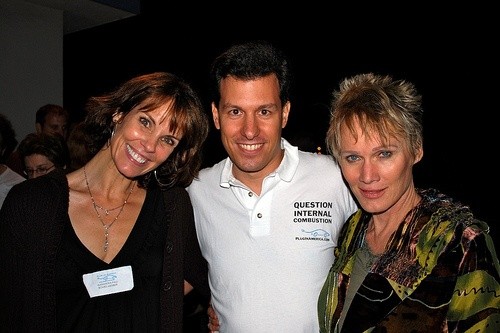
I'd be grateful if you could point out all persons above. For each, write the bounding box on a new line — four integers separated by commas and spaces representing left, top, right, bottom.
1, 71, 211, 332
35, 104, 68, 138
68, 123, 110, 171
16, 133, 67, 179
0, 114, 28, 209
208, 72, 500, 332
185, 47, 360, 332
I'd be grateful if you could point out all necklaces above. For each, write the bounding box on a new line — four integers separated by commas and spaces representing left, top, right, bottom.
83, 164, 136, 252
